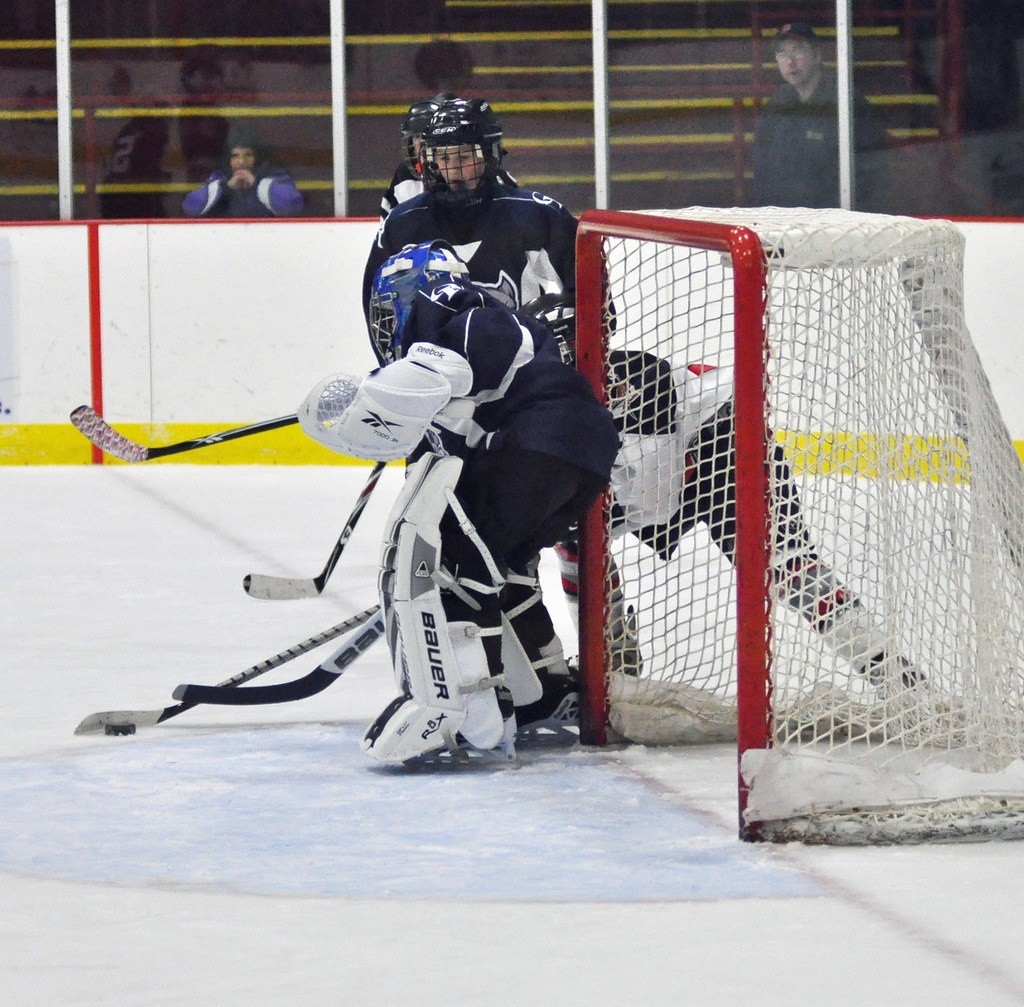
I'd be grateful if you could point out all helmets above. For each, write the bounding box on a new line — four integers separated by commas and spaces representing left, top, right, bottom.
368, 239, 469, 361
401, 91, 456, 180
419, 97, 503, 210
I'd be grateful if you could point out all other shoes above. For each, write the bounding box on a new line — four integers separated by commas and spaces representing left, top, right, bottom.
562, 605, 643, 676
866, 657, 923, 698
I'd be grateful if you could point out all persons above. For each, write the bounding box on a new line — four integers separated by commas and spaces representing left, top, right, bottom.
306, 240, 616, 764
522, 293, 930, 699
365, 92, 616, 386
753, 24, 887, 207
181, 125, 303, 219
100, 101, 173, 219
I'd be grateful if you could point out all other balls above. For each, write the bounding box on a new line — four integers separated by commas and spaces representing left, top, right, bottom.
104, 722, 137, 737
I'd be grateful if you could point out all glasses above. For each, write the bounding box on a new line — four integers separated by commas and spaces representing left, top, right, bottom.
775, 44, 810, 60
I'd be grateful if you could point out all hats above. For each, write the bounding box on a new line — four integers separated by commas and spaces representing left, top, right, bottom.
773, 21, 817, 48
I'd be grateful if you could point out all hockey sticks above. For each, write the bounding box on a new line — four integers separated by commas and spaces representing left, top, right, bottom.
239, 462, 386, 601
69, 403, 353, 463
171, 606, 388, 707
72, 602, 382, 738
